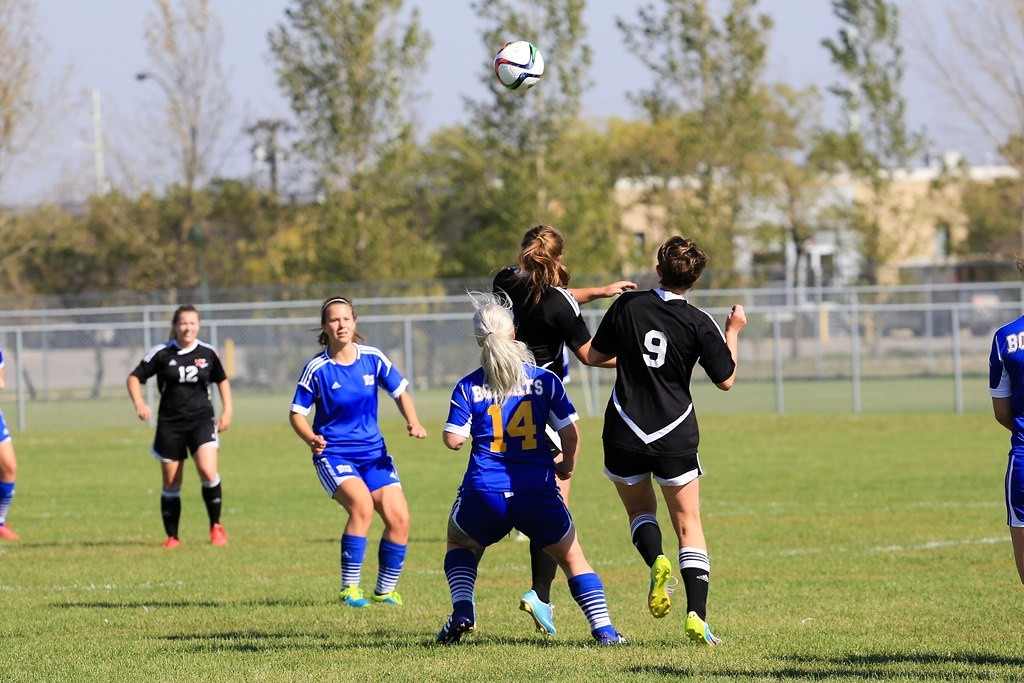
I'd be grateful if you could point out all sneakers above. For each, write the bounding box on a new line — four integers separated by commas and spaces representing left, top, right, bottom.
647, 555, 672, 620
0, 523, 21, 543
162, 536, 180, 550
436, 604, 474, 646
519, 590, 556, 641
595, 631, 630, 645
338, 584, 371, 608
371, 591, 403, 607
210, 523, 226, 546
683, 611, 723, 648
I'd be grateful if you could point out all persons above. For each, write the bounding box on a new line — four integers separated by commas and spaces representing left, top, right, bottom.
493, 225, 638, 636
289, 296, 427, 607
0, 350, 21, 541
989, 254, 1024, 585
588, 237, 747, 647
435, 293, 627, 646
127, 304, 232, 550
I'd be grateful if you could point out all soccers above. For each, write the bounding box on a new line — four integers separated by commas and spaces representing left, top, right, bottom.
492, 39, 546, 94
